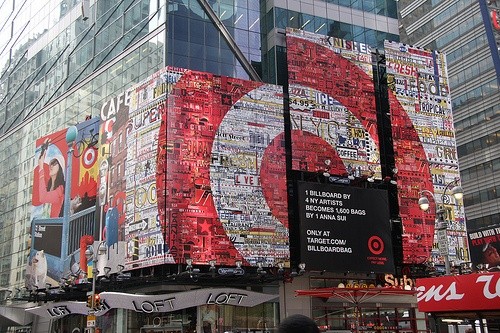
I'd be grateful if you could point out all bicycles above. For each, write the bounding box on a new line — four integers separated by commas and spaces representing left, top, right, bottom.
73, 128, 99, 158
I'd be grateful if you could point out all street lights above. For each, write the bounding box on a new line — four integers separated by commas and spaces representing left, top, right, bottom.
84, 239, 107, 333
58, 126, 78, 276
418, 177, 464, 276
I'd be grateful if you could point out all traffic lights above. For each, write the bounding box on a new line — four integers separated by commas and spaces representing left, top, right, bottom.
86, 293, 100, 311
